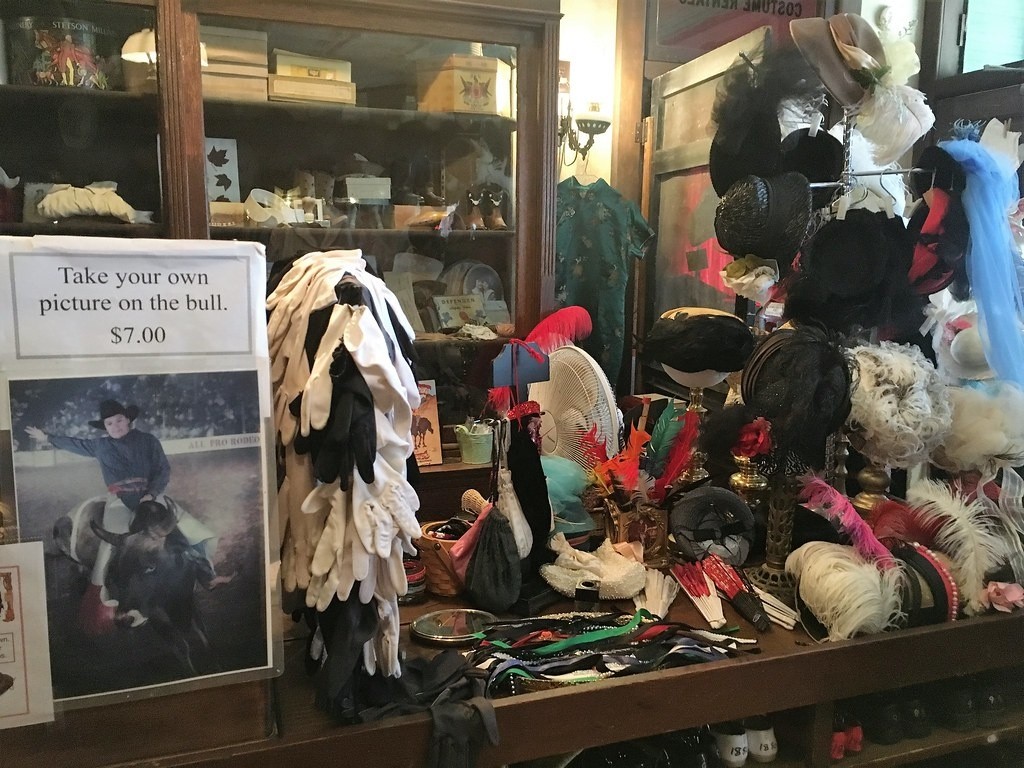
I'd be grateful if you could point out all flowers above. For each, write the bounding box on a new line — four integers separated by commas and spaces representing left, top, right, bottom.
879, 30, 921, 86
720, 253, 780, 304
941, 319, 972, 346
731, 418, 772, 460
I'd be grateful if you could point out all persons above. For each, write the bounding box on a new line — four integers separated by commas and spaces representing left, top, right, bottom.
23, 399, 237, 622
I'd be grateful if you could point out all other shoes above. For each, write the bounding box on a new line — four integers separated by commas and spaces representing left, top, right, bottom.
830, 705, 862, 761
663, 729, 725, 768
917, 677, 1008, 732
709, 716, 779, 768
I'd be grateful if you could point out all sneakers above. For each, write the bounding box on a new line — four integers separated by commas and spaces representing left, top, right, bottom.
847, 691, 932, 745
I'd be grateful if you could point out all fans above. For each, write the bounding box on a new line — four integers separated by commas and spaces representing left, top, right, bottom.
529, 346, 626, 472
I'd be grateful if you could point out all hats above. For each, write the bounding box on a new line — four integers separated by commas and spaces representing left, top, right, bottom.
708, 12, 954, 469
86, 399, 140, 430
670, 485, 756, 566
931, 300, 1024, 381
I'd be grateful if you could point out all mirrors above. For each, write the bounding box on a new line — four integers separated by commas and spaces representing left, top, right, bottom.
409, 608, 498, 645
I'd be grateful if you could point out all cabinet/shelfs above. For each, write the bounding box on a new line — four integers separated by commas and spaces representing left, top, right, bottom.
0, 0, 564, 519
0, 561, 1024, 768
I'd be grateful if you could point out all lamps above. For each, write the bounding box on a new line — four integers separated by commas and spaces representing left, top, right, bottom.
557, 89, 610, 162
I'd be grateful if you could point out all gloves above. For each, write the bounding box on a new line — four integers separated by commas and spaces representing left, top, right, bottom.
428, 674, 499, 768
360, 648, 469, 723
267, 248, 422, 679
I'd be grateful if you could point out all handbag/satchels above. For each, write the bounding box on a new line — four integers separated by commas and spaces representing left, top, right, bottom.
464, 507, 523, 614
489, 466, 533, 560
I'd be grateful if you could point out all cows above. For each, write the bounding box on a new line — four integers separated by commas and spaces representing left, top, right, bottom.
51, 494, 229, 692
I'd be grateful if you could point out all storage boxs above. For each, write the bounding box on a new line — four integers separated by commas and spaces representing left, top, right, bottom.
199, 25, 358, 106
345, 177, 392, 199
209, 201, 257, 228
383, 204, 447, 230
406, 53, 511, 117
